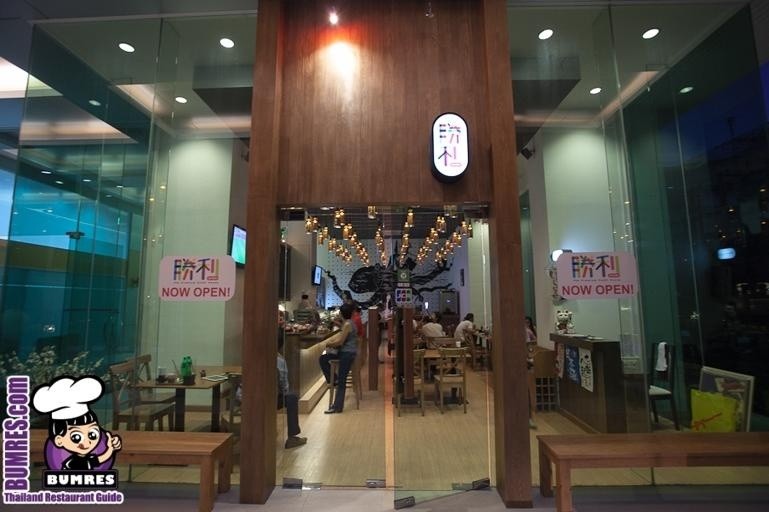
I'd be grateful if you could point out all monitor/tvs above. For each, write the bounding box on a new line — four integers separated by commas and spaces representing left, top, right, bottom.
229, 224, 246, 268
312, 264, 322, 286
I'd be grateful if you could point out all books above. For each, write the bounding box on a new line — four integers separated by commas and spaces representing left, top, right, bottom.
698, 365, 755, 433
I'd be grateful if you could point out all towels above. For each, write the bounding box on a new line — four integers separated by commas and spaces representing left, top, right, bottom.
655, 342, 668, 371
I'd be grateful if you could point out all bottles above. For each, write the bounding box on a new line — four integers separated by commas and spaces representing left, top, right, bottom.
181, 356, 192, 381
201, 370, 206, 377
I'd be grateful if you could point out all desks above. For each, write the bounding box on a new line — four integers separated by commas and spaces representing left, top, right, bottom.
131, 365, 243, 432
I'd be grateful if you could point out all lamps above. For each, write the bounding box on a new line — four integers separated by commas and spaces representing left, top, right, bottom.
305, 205, 473, 267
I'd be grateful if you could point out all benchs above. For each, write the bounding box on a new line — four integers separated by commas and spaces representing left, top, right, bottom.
29, 430, 234, 512
537, 431, 769, 512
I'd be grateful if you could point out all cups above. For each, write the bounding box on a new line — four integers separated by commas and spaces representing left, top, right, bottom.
167, 373, 176, 383
158, 366, 166, 383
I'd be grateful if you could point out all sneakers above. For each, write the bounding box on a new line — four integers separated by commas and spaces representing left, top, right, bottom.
324, 406, 342, 413
285, 436, 307, 448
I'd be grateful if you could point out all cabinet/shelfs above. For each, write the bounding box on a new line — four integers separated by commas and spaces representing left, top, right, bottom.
528, 372, 560, 412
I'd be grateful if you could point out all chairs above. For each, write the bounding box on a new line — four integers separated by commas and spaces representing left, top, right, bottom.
110, 362, 175, 431
221, 373, 242, 472
328, 329, 491, 417
129, 354, 178, 404
646, 343, 679, 431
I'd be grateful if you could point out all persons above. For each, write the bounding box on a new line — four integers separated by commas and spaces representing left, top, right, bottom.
524, 316, 537, 344
413, 314, 422, 331
232, 327, 308, 448
316, 303, 358, 414
419, 317, 446, 350
330, 299, 364, 348
452, 312, 475, 344
430, 311, 443, 326
